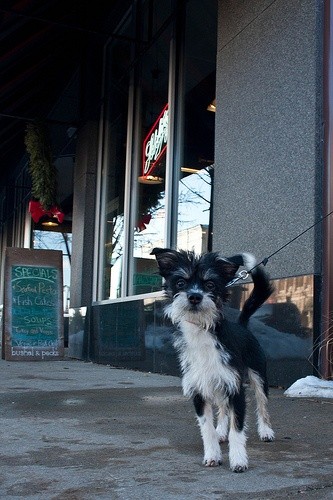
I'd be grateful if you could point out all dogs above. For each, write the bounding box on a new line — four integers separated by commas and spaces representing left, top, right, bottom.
149, 247, 276, 473
